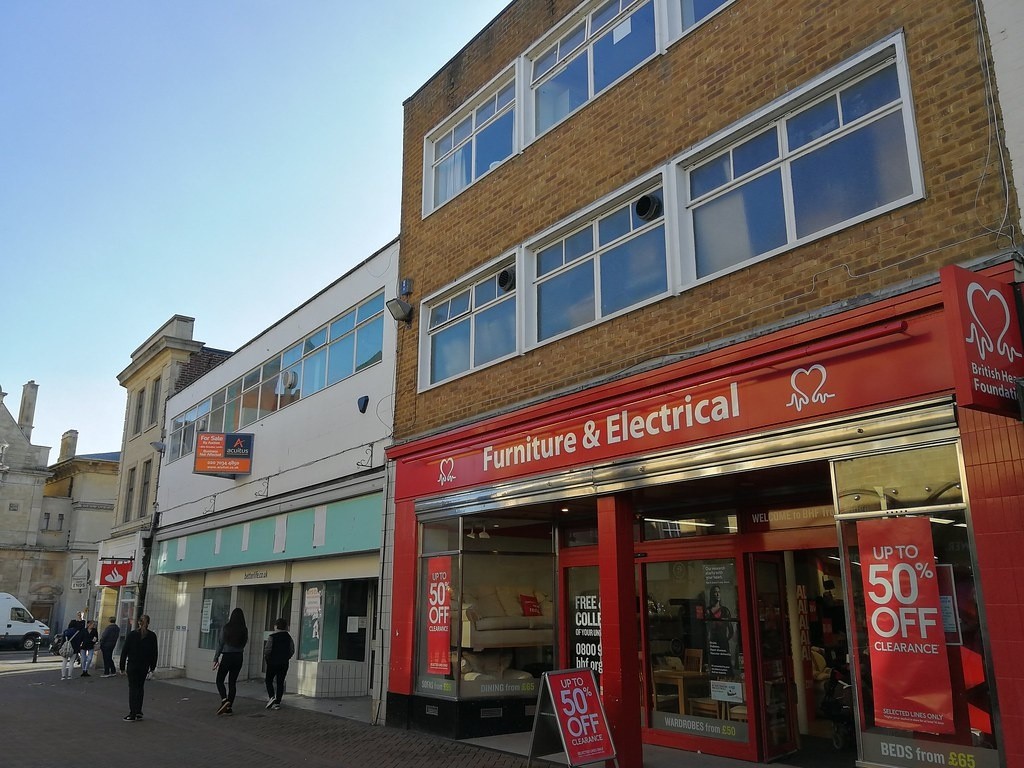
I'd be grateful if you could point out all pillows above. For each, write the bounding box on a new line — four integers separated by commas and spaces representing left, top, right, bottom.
519, 594, 544, 617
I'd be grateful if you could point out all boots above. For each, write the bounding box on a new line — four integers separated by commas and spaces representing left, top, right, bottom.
81, 670, 85, 677
86, 669, 91, 676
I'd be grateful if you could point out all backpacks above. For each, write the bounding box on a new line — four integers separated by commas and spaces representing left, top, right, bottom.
59, 630, 80, 658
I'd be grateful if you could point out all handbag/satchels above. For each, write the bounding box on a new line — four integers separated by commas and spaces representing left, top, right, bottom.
94, 642, 101, 651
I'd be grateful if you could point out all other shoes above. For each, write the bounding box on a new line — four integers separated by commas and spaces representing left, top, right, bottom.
67, 677, 72, 679
61, 677, 65, 680
99, 674, 109, 677
109, 674, 116, 676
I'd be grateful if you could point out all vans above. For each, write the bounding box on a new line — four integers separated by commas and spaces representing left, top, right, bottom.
0, 592, 51, 650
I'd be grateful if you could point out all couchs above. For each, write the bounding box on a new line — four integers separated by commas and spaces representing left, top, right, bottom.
450, 585, 553, 652
450, 651, 534, 681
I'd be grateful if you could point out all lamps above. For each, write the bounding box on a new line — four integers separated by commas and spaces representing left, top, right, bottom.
386, 298, 414, 324
466, 528, 476, 539
479, 529, 490, 539
149, 442, 165, 455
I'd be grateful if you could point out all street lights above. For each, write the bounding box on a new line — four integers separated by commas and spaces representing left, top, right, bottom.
124, 441, 167, 628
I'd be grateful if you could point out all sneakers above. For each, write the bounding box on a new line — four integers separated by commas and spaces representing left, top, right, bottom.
223, 708, 232, 715
215, 699, 231, 715
136, 714, 144, 720
123, 715, 135, 721
265, 696, 276, 708
271, 704, 280, 710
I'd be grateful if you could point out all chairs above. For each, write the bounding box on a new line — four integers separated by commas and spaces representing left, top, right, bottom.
637, 648, 747, 723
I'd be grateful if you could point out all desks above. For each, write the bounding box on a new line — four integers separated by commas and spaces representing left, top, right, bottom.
653, 669, 707, 716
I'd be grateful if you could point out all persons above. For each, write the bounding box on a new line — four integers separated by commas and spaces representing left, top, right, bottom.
264, 618, 295, 710
120, 615, 158, 721
212, 608, 248, 716
100, 616, 120, 678
59, 610, 98, 679
706, 585, 734, 677
